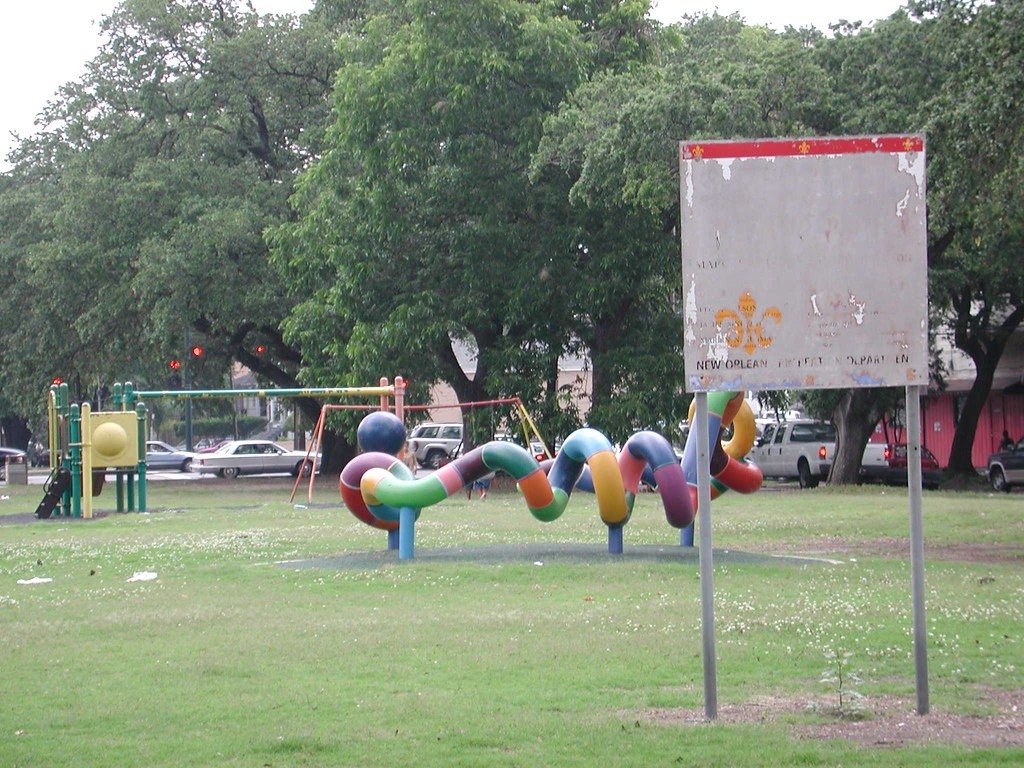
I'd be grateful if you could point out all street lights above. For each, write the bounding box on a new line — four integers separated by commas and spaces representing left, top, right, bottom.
168, 346, 203, 453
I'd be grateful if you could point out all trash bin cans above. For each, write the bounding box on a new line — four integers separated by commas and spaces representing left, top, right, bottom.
5, 455, 27, 485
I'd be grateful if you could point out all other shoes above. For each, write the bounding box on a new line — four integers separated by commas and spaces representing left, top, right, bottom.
480, 493, 486, 502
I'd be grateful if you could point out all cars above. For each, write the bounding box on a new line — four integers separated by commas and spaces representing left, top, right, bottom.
889, 443, 940, 470
0, 447, 28, 480
403, 421, 559, 471
646, 416, 891, 489
196, 439, 322, 480
987, 435, 1024, 493
144, 439, 200, 473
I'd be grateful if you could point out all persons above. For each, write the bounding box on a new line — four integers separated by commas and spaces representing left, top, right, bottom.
404, 442, 417, 475
459, 441, 495, 501
